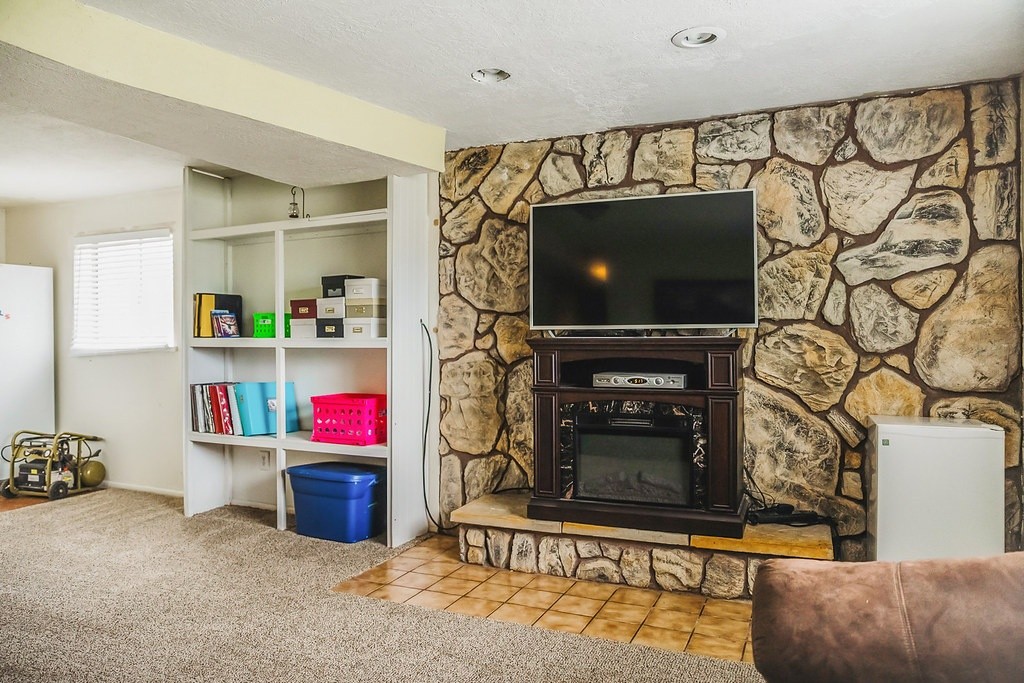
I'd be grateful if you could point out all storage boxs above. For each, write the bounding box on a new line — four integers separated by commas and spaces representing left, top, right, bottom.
286, 462, 386, 545
251, 312, 291, 338
288, 274, 385, 338
310, 393, 386, 445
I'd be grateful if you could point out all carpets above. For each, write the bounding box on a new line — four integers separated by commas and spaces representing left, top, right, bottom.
0, 487, 762, 683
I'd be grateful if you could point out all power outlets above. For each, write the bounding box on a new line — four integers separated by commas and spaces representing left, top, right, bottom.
259, 450, 270, 472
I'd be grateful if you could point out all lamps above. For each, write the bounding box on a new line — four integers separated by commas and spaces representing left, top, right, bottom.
288, 185, 307, 219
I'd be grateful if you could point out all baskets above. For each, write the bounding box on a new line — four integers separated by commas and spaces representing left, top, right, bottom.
310, 393, 387, 446
253, 312, 291, 338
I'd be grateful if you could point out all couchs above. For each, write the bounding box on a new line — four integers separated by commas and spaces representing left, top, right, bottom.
751, 550, 1024, 683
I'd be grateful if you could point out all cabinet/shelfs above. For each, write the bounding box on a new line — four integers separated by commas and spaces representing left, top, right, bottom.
183, 166, 440, 551
523, 337, 750, 539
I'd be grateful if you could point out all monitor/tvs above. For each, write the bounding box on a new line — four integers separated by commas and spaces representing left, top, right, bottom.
530, 188, 758, 338
